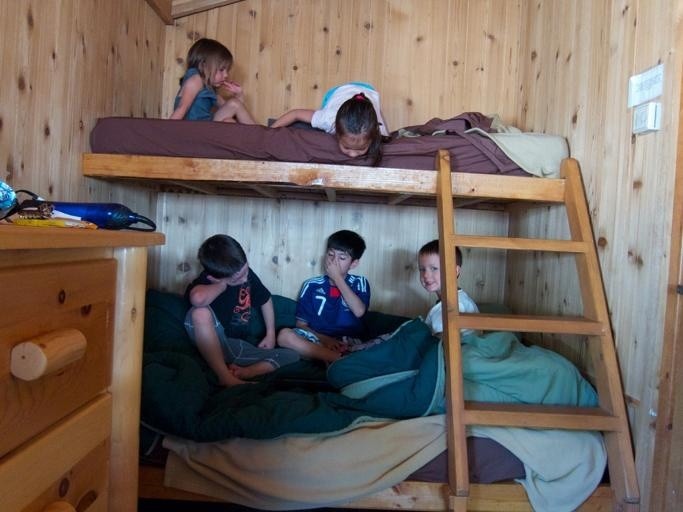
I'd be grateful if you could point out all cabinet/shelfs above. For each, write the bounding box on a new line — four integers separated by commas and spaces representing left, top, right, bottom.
0, 222, 168, 512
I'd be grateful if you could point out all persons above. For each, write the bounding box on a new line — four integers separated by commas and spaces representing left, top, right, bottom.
182, 233, 302, 389
347, 238, 484, 355
167, 37, 258, 126
268, 80, 392, 159
276, 228, 396, 365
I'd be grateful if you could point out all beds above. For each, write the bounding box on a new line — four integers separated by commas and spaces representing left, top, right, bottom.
82, 110, 640, 512
